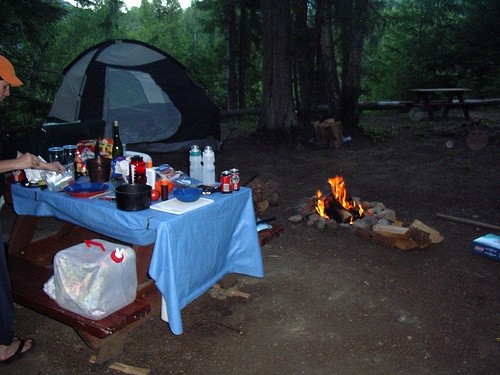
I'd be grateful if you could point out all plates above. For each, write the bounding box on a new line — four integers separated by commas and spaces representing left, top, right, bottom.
64, 183, 108, 197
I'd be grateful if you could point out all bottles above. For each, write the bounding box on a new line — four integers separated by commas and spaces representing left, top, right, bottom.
128, 154, 148, 184
112, 120, 123, 158
189, 145, 203, 181
202, 146, 216, 183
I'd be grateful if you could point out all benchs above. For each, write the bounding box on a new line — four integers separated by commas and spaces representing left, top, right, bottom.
399, 101, 477, 112
4, 252, 151, 339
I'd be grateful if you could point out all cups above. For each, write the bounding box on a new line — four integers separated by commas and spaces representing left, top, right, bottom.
63, 145, 79, 162
48, 147, 64, 164
86, 157, 112, 182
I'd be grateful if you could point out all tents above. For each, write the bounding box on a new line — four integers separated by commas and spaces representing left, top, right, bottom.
41, 37, 222, 154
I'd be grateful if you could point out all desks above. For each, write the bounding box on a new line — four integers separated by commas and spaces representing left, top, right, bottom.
4, 177, 266, 375
407, 88, 473, 121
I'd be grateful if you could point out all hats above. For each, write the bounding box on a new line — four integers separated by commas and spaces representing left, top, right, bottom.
0, 55, 23, 87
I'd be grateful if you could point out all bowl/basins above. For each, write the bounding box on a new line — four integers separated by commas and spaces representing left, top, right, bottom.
114, 183, 151, 211
172, 187, 201, 202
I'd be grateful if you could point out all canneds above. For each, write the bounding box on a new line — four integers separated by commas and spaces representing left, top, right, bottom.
220, 171, 233, 194
229, 168, 240, 191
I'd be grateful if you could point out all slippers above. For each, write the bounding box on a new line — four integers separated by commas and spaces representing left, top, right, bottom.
0, 338, 34, 363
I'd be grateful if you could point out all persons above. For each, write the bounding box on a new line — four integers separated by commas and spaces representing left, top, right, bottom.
0, 54, 64, 366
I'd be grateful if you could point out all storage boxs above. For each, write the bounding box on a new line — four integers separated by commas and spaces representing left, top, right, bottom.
471, 233, 500, 261
